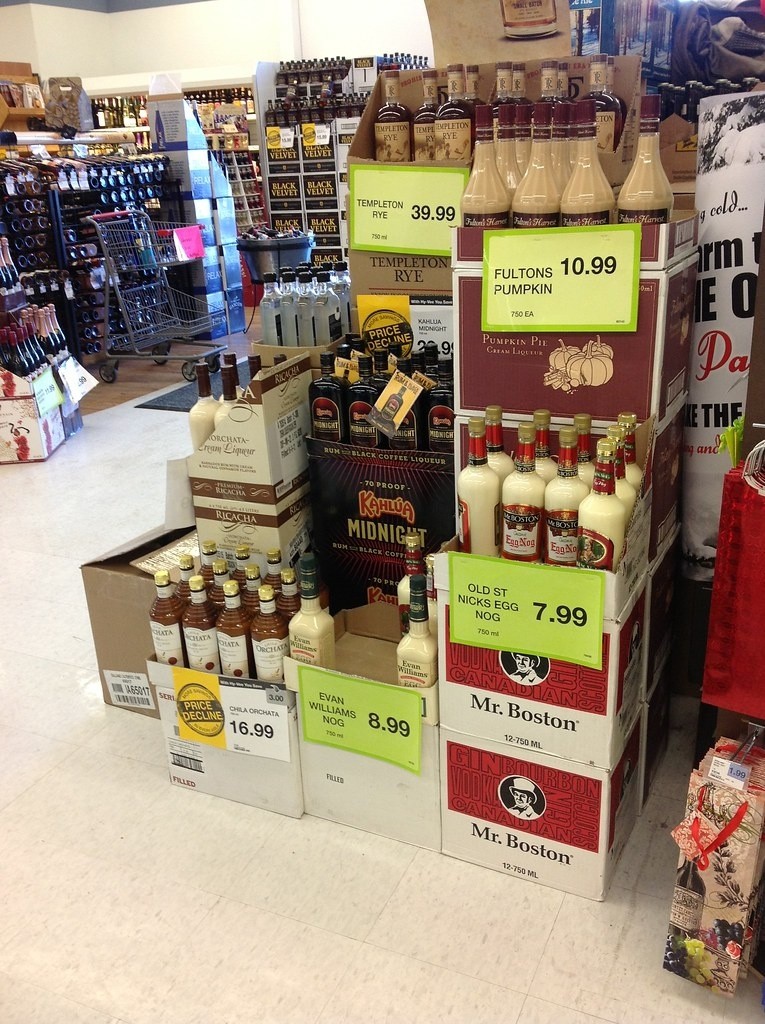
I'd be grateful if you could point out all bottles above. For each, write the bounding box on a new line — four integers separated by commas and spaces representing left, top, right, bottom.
377, 385, 407, 424
373, 53, 675, 226
148, 530, 439, 688
454, 406, 643, 572
0, 86, 255, 379
500, 0, 559, 40
668, 857, 707, 941
240, 225, 306, 241
265, 51, 370, 127
187, 260, 454, 454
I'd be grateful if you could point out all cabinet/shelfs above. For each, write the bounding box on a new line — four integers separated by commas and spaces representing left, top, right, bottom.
213, 150, 270, 235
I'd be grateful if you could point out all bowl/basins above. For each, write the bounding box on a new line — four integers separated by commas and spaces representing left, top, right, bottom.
236, 239, 315, 283
686, 929, 740, 996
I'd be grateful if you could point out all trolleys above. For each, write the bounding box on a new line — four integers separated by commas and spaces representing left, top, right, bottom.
82, 208, 231, 383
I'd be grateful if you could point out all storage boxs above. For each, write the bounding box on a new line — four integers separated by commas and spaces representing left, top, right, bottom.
1, 53, 700, 902
